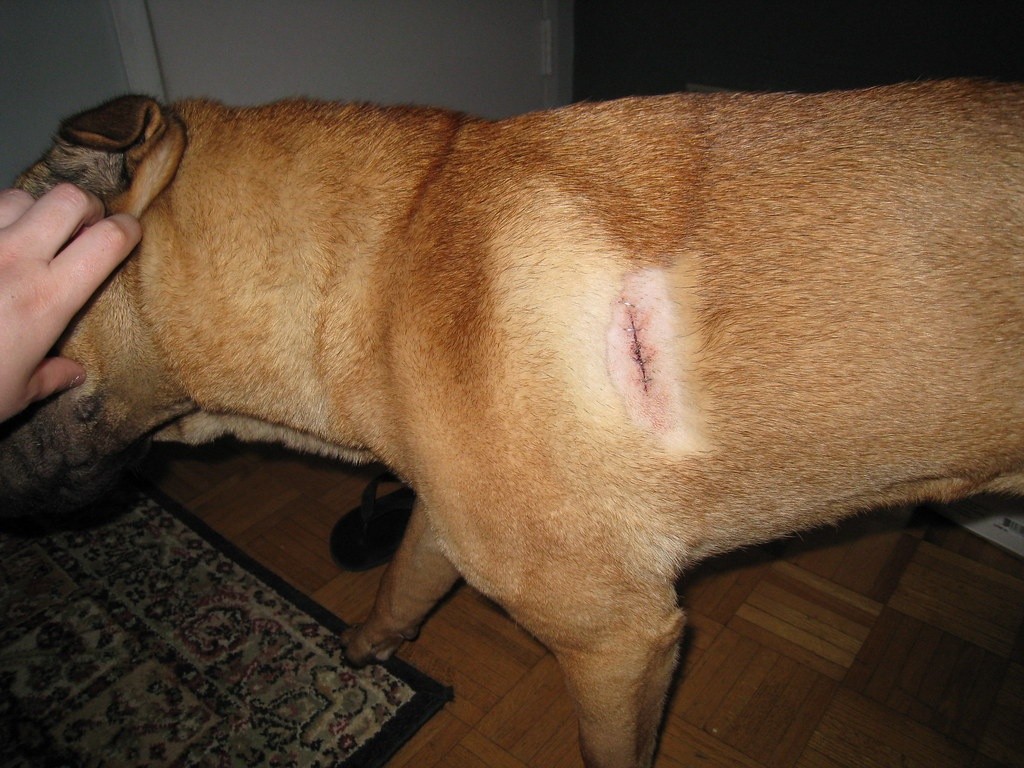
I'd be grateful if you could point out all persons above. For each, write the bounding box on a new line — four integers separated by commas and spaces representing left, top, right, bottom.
0, 182, 143, 424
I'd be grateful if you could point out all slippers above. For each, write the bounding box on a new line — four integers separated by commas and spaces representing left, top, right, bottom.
331, 471, 416, 571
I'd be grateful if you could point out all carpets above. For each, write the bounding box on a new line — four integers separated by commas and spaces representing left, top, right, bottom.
0, 484, 454, 768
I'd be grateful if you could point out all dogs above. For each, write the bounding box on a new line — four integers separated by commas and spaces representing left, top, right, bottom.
0, 79, 1024, 768
